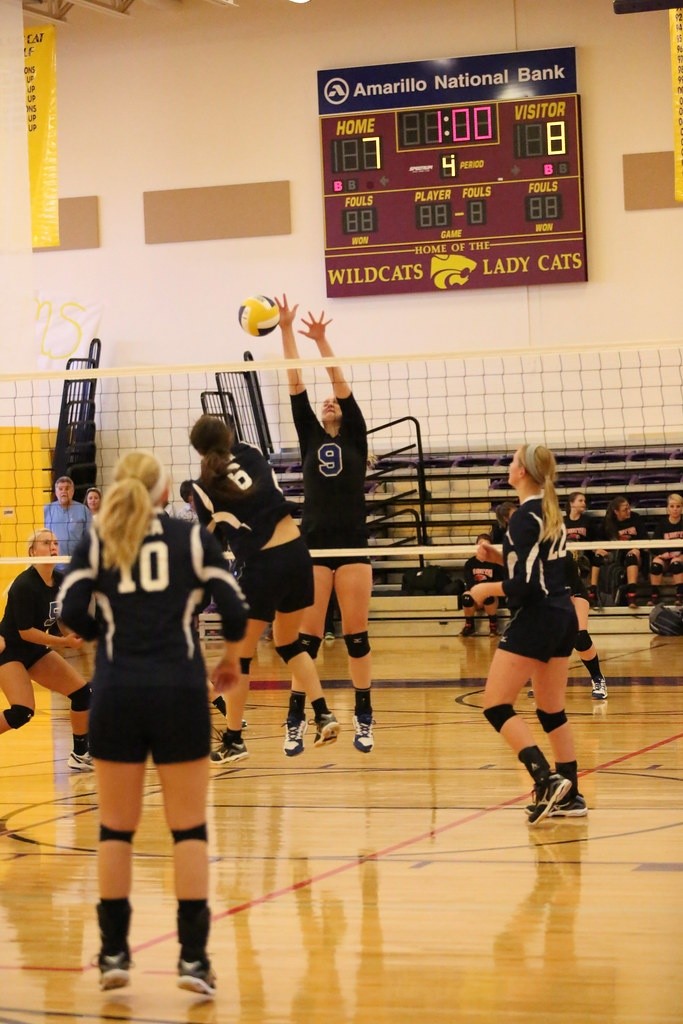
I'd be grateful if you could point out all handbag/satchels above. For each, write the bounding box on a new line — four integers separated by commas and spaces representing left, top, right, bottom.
402, 564, 452, 590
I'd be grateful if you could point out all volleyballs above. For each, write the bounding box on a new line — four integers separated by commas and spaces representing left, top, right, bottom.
238, 294, 282, 337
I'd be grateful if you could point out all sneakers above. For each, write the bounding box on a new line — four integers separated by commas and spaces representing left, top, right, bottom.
210, 742, 248, 764
178, 958, 218, 995
67, 750, 95, 771
459, 622, 476, 636
591, 674, 608, 699
98, 950, 131, 992
527, 689, 534, 698
528, 773, 572, 825
489, 622, 498, 636
524, 794, 588, 817
352, 712, 375, 753
284, 718, 307, 756
313, 711, 340, 748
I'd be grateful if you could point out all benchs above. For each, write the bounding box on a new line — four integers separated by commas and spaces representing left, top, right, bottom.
270, 442, 683, 618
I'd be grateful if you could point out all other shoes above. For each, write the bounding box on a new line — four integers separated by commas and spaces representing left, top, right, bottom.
587, 593, 598, 608
647, 594, 658, 606
674, 593, 683, 606
628, 593, 637, 607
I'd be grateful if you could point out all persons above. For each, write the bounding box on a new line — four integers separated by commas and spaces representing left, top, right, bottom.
0, 529, 96, 771
174, 417, 338, 764
44, 477, 103, 574
470, 444, 587, 826
52, 451, 251, 997
459, 533, 505, 638
528, 551, 609, 699
491, 492, 683, 608
270, 293, 374, 754
